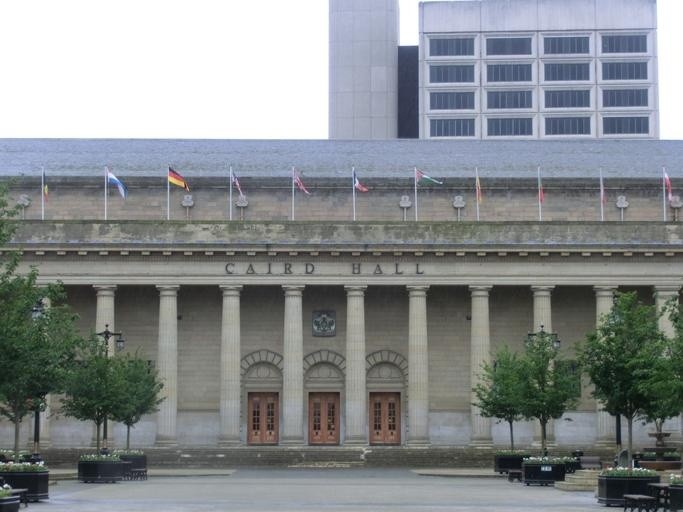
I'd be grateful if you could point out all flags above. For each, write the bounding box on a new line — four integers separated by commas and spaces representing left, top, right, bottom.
352, 168, 369, 192
43, 170, 48, 202
107, 169, 128, 200
665, 173, 672, 200
476, 177, 482, 204
232, 168, 243, 201
169, 167, 189, 192
294, 169, 313, 196
416, 169, 443, 186
539, 176, 544, 205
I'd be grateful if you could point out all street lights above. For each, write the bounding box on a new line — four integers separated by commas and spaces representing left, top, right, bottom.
88, 323, 124, 449
32, 298, 46, 454
526, 324, 560, 456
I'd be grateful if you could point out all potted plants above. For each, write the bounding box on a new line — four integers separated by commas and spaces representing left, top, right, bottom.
1, 175, 82, 512
580, 290, 681, 509
469, 328, 580, 489
61, 346, 166, 486
